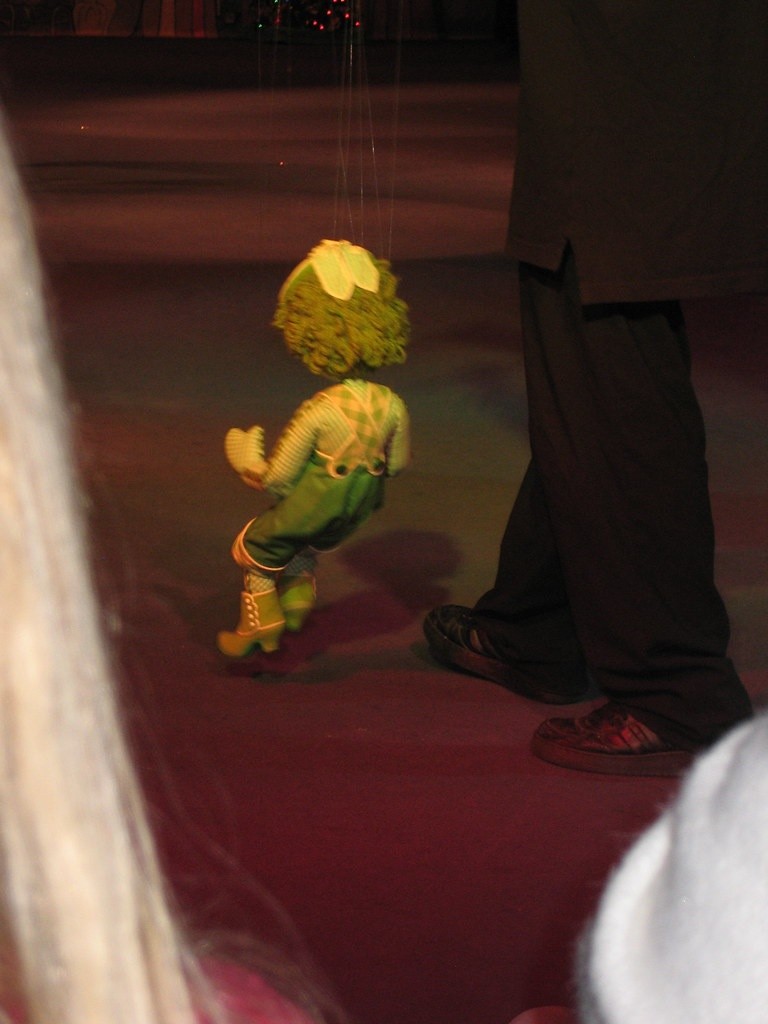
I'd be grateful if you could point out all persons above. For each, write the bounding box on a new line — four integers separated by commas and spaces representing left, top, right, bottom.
422, 1, 768, 775
215, 236, 414, 660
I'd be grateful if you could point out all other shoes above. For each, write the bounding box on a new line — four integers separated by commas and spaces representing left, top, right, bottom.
423, 604, 602, 703
531, 697, 701, 774
